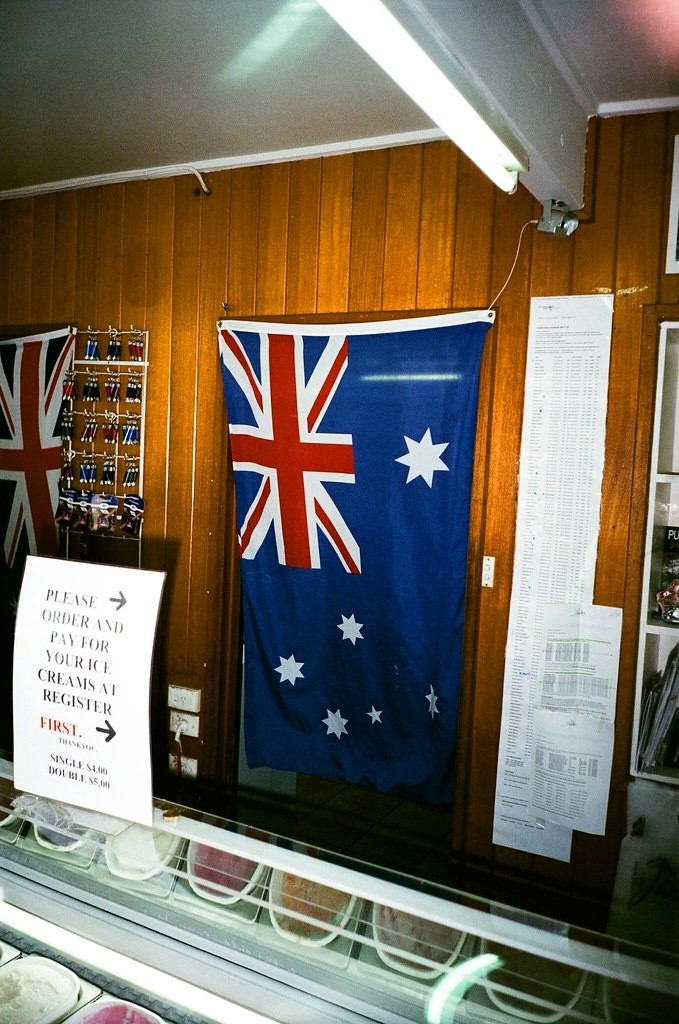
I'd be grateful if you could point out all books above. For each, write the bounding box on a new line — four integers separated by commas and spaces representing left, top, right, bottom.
657, 526, 679, 626
635, 642, 679, 773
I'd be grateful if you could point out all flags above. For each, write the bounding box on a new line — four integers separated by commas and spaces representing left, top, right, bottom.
1, 321, 77, 608
216, 309, 501, 808
